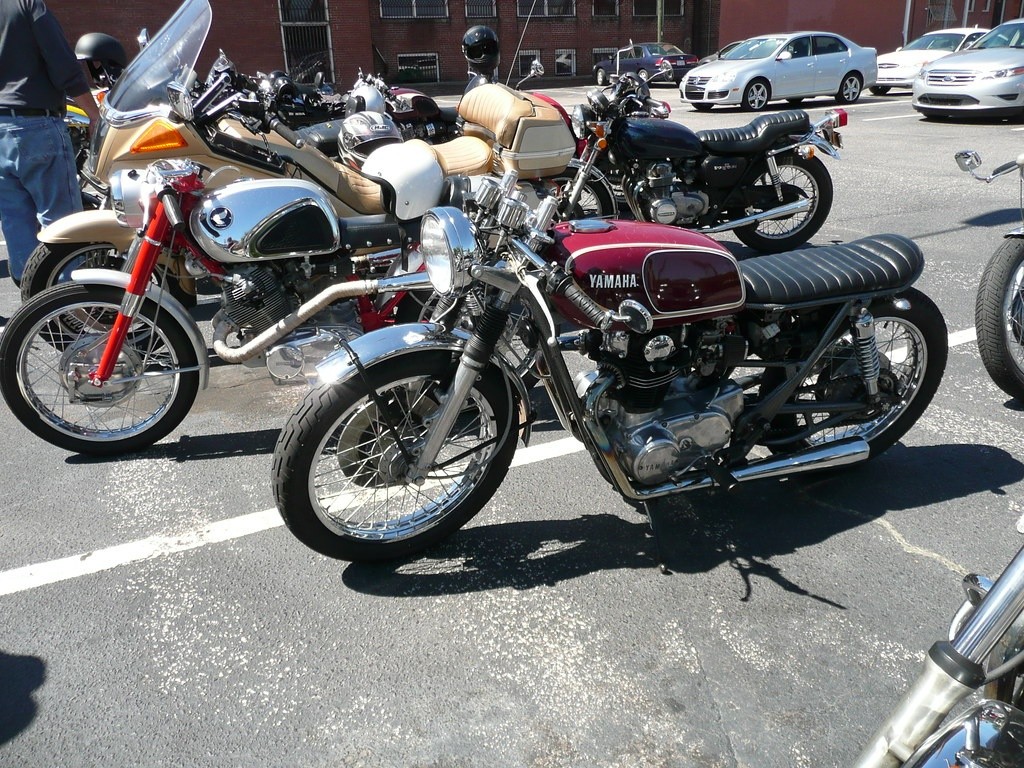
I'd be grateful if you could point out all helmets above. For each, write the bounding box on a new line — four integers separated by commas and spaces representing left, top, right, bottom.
359, 138, 444, 220
338, 111, 403, 172
267, 70, 286, 86
75, 32, 127, 68
349, 85, 386, 113
273, 77, 296, 98
461, 25, 502, 73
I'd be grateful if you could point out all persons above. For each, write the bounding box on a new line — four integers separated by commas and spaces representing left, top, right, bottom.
0, 0, 100, 285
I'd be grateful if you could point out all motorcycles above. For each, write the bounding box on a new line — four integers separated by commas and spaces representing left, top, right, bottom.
0, 0, 1024, 768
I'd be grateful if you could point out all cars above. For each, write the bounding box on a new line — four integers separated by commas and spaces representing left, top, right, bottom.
912, 18, 1024, 121
591, 41, 699, 88
867, 26, 1012, 96
677, 30, 879, 113
696, 38, 793, 66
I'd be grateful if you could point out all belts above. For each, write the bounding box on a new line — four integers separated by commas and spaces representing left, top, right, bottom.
0, 106, 64, 118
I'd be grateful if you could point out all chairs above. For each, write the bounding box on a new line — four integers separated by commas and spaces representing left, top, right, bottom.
792, 40, 804, 58
826, 44, 840, 52
